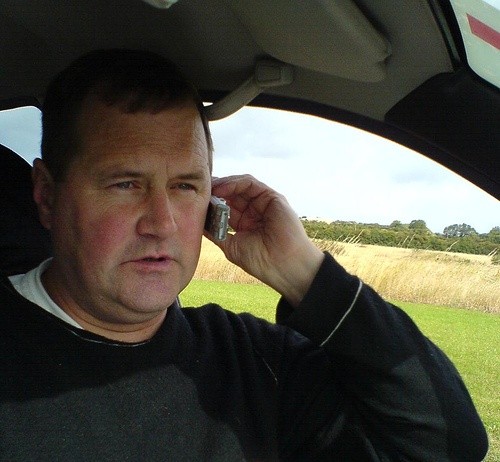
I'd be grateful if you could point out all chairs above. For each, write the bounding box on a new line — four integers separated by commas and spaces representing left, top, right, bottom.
0, 141, 49, 277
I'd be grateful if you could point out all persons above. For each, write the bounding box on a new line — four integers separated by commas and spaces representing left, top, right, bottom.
0, 49, 491, 462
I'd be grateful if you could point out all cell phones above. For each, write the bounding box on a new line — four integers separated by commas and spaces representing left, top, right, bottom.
203, 193, 230, 241
